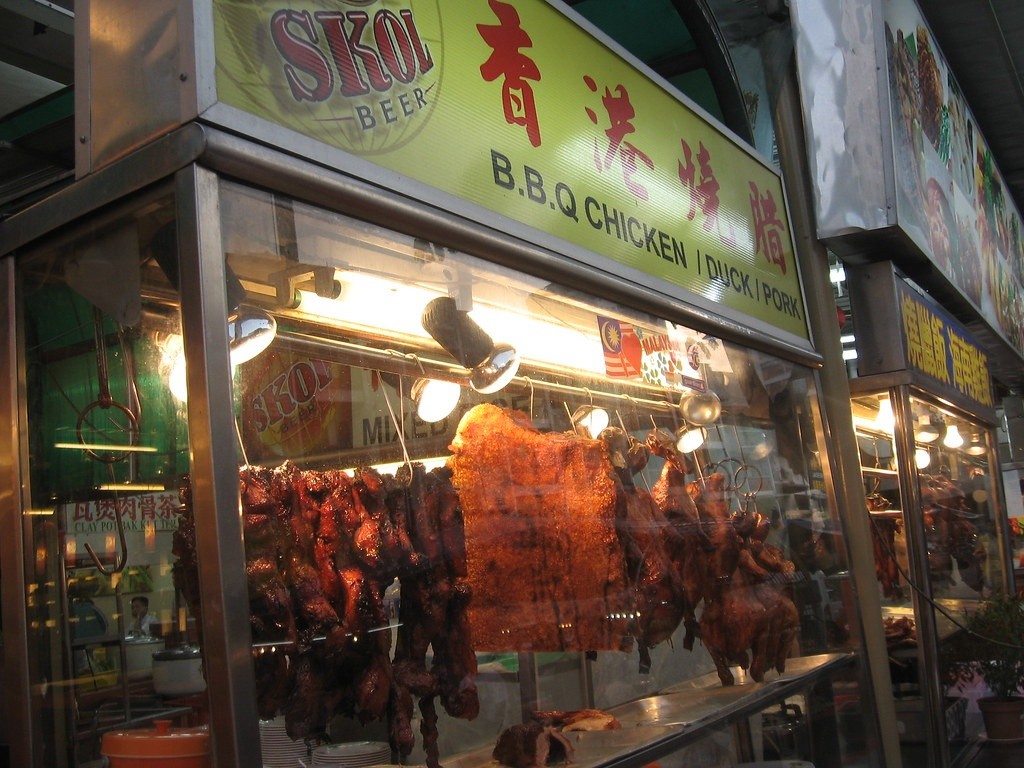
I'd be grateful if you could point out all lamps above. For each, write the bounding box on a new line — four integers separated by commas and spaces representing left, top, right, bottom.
269, 264, 606, 375
423, 273, 519, 394
966, 426, 986, 455
379, 371, 461, 422
570, 405, 611, 438
150, 218, 277, 364
660, 417, 708, 454
942, 414, 963, 448
913, 400, 939, 442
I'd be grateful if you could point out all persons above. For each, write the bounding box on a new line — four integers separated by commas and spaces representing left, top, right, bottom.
127, 596, 158, 635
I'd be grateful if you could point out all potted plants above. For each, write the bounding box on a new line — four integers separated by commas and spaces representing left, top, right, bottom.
958, 579, 1024, 740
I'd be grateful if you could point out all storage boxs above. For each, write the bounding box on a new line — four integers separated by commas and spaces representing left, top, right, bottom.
894, 683, 947, 744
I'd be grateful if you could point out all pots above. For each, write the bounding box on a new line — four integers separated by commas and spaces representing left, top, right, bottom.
151, 643, 207, 694
103, 629, 165, 679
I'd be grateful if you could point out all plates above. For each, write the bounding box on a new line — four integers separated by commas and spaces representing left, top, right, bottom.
258, 716, 392, 768
